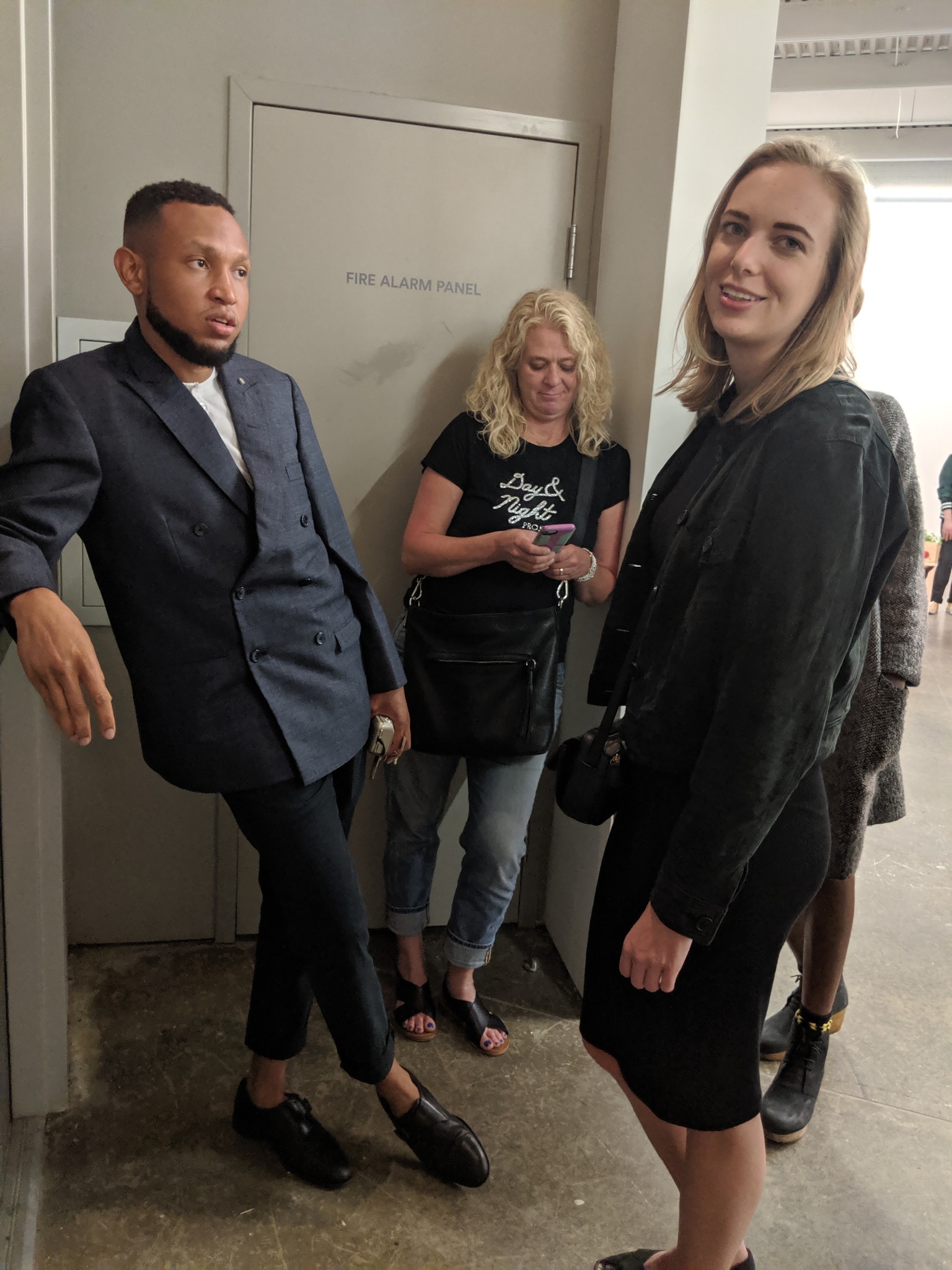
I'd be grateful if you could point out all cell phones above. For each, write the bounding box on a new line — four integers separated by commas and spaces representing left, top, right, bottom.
532, 524, 577, 553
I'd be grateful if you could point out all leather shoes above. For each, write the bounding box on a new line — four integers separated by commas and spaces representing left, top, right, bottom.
373, 1063, 489, 1187
230, 1075, 352, 1187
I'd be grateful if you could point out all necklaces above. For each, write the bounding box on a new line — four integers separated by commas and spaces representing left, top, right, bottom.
523, 428, 568, 447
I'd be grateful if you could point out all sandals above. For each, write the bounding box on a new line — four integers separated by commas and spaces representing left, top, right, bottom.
392, 960, 438, 1043
439, 974, 511, 1057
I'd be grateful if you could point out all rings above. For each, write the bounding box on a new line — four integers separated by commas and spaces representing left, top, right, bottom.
560, 568, 564, 575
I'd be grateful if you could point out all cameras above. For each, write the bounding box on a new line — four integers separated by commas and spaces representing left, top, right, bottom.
368, 716, 395, 755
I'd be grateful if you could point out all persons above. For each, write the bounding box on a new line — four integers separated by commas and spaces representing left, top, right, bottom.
578, 135, 913, 1270
927, 453, 952, 614
0, 179, 492, 1192
758, 280, 929, 1146
380, 285, 632, 1057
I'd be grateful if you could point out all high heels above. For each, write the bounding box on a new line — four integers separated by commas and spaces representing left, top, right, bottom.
763, 975, 849, 1063
761, 1020, 830, 1142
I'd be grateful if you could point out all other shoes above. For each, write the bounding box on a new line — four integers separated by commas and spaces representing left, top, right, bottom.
928, 602, 938, 614
946, 605, 952, 614
592, 1248, 756, 1270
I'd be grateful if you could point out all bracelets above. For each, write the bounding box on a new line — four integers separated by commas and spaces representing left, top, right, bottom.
574, 548, 597, 582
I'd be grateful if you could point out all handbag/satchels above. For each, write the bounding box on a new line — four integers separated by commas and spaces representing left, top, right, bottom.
425, 655, 537, 765
547, 717, 625, 827
